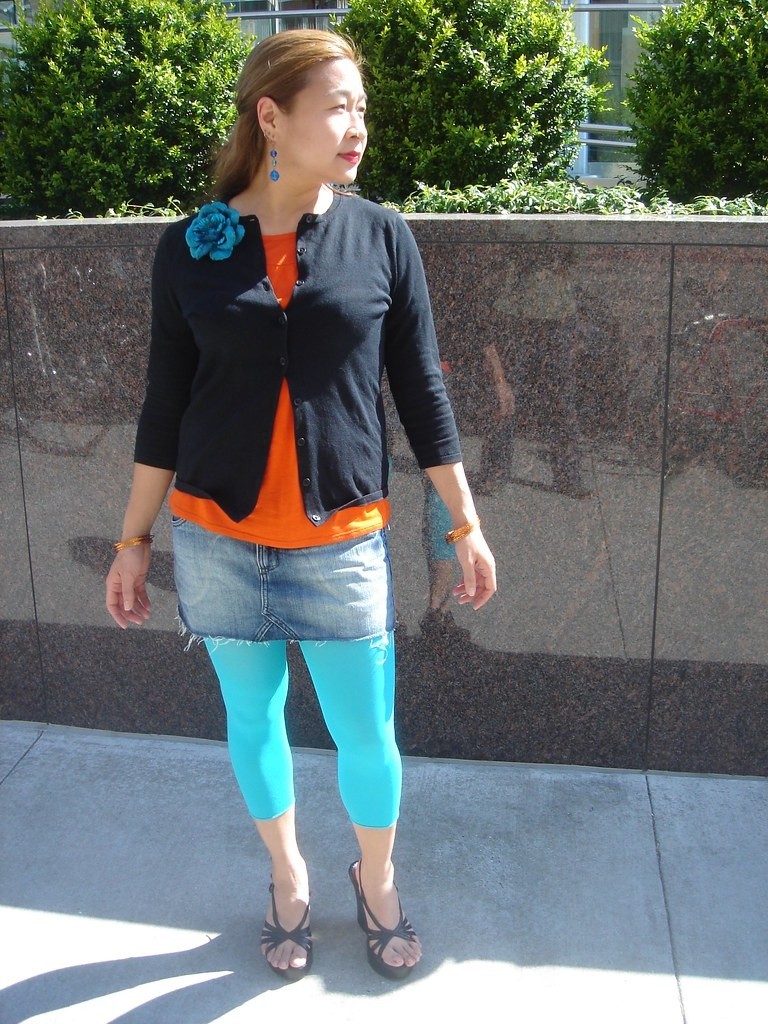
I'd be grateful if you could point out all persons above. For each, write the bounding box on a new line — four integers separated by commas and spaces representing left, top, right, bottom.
106, 28, 501, 978
387, 244, 595, 644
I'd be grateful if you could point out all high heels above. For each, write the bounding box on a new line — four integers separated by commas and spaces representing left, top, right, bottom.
260, 872, 312, 982
349, 857, 417, 980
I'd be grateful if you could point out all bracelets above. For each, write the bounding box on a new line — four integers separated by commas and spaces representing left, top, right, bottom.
445, 516, 482, 544
114, 534, 154, 553
